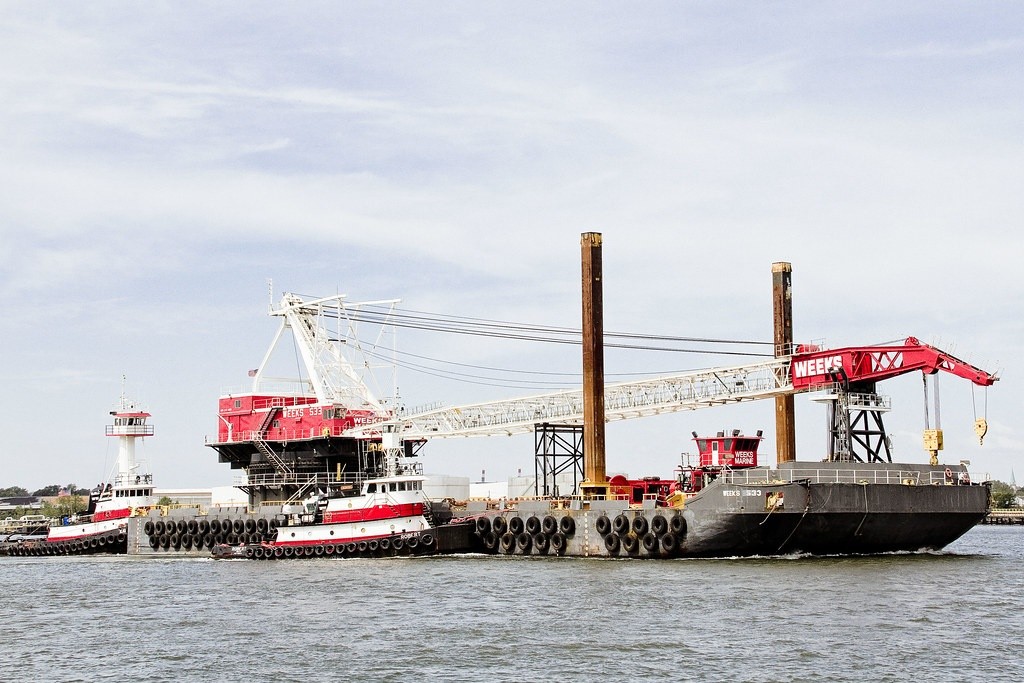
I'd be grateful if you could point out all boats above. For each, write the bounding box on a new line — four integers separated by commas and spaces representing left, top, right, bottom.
0, 279, 1000, 558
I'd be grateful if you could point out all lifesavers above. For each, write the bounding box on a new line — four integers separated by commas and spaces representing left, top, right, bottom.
595, 515, 686, 553
244, 533, 434, 558
322, 427, 330, 436
944, 467, 953, 478
7, 533, 126, 556
664, 486, 668, 492
476, 515, 575, 550
105, 510, 111, 518
144, 519, 281, 548
